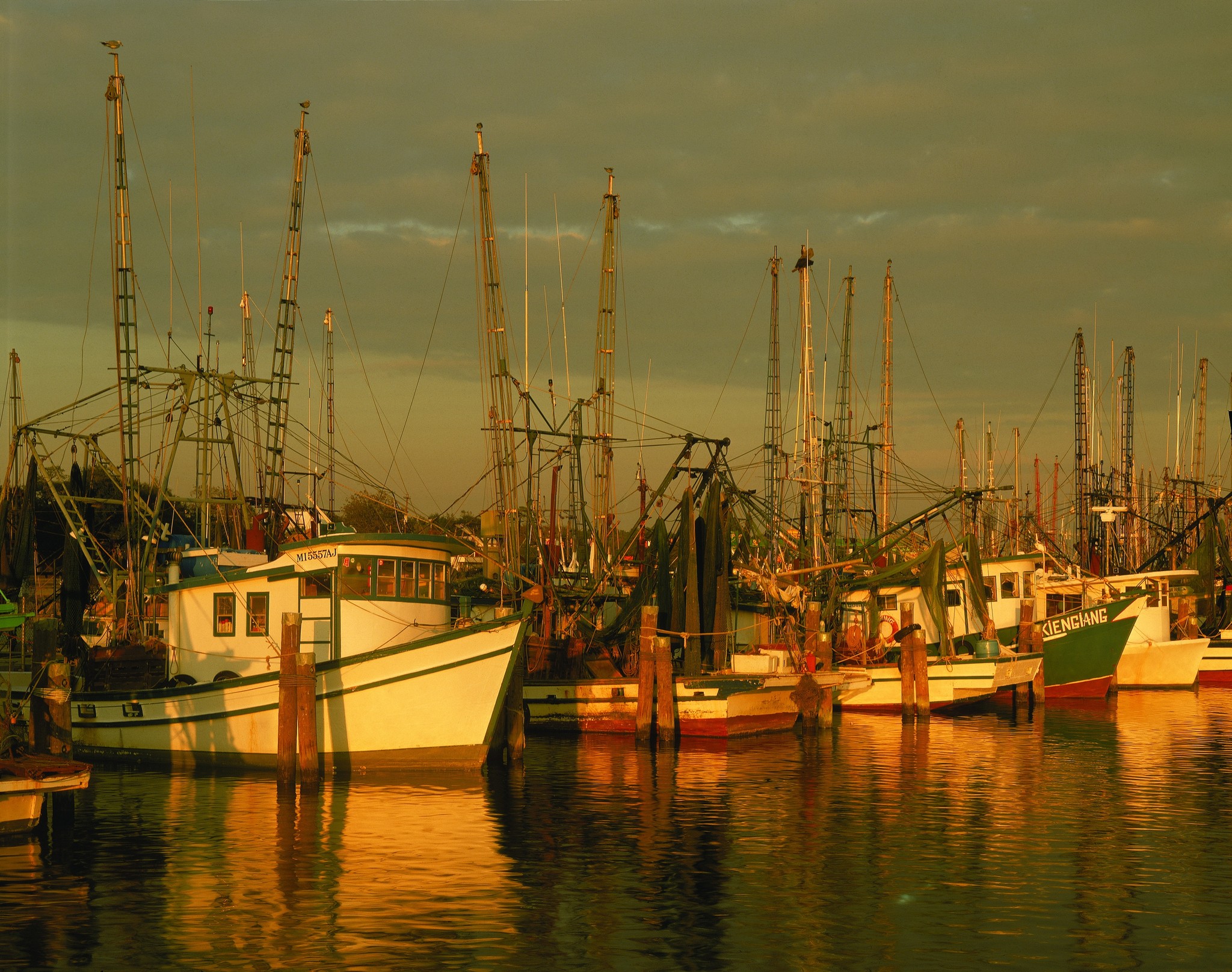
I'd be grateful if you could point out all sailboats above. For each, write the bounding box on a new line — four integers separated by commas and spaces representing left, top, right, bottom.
1, 37, 1232, 845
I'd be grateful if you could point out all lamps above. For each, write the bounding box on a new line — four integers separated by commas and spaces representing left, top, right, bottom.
142, 532, 157, 544
69, 520, 86, 542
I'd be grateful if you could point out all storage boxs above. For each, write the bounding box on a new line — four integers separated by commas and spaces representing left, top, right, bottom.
731, 648, 800, 673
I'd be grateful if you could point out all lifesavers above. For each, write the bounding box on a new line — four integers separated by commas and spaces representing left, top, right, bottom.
1055, 596, 1071, 614
167, 670, 240, 687
880, 615, 898, 643
955, 640, 974, 655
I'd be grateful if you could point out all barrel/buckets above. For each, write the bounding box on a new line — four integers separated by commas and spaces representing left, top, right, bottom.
155, 535, 262, 578
504, 563, 547, 590
976, 639, 1001, 658
